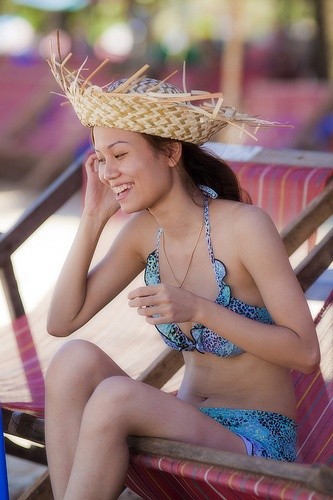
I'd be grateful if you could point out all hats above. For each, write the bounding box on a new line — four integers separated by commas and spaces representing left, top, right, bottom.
42, 29, 293, 147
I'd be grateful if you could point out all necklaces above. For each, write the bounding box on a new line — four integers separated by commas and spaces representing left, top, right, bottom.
162, 219, 205, 289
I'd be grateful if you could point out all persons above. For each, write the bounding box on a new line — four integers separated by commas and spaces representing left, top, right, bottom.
44, 76, 321, 500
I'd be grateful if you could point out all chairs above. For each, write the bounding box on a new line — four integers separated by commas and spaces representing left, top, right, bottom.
0, 130, 333, 500
